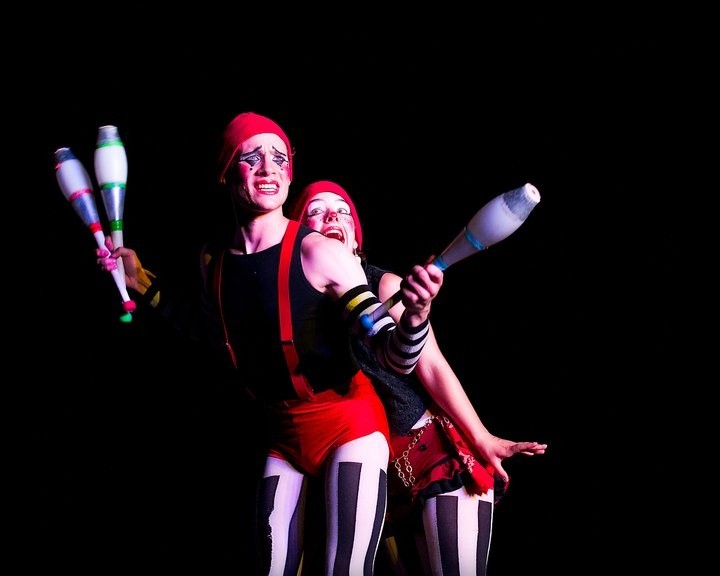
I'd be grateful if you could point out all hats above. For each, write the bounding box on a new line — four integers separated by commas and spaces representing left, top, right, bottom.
216, 112, 292, 184
291, 180, 363, 251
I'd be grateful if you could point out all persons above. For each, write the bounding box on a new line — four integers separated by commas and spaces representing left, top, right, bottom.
94, 111, 444, 576
291, 180, 548, 576
